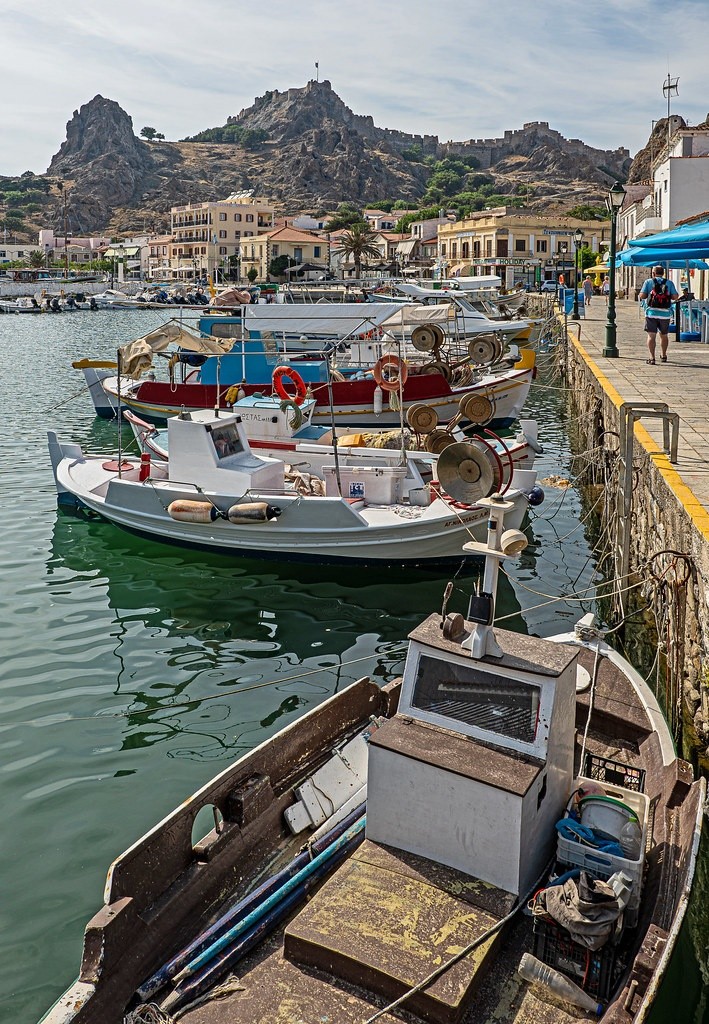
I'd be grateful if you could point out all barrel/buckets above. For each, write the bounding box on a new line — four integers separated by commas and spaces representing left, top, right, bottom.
582, 800, 638, 840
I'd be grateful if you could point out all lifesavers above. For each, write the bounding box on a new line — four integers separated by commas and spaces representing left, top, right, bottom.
374, 355, 408, 391
559, 275, 564, 285
273, 366, 306, 405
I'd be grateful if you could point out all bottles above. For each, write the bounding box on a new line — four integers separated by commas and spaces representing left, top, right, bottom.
517, 952, 603, 1016
618, 815, 643, 862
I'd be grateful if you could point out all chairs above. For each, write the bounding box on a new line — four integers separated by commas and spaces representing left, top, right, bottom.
637, 295, 709, 344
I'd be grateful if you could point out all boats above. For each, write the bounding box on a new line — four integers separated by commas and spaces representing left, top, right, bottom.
0, 275, 539, 437
44, 392, 546, 564
33, 428, 705, 1024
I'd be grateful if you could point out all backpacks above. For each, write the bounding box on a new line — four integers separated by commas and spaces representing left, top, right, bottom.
646, 277, 672, 309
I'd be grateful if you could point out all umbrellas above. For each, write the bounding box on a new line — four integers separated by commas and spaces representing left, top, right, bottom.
626, 219, 708, 246
606, 260, 709, 289
618, 247, 709, 301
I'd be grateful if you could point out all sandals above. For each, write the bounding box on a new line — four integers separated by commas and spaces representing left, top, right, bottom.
660, 354, 667, 362
646, 358, 656, 365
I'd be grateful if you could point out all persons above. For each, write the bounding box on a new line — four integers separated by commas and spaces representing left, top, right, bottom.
639, 265, 679, 365
13, 280, 274, 315
601, 276, 611, 306
674, 288, 695, 302
582, 276, 593, 305
559, 272, 565, 287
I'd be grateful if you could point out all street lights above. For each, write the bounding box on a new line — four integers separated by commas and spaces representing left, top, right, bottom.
603, 181, 625, 359
520, 240, 567, 307
571, 227, 583, 321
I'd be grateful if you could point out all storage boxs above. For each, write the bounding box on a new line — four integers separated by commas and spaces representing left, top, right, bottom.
579, 751, 646, 795
555, 774, 650, 929
532, 915, 636, 1001
319, 466, 408, 505
